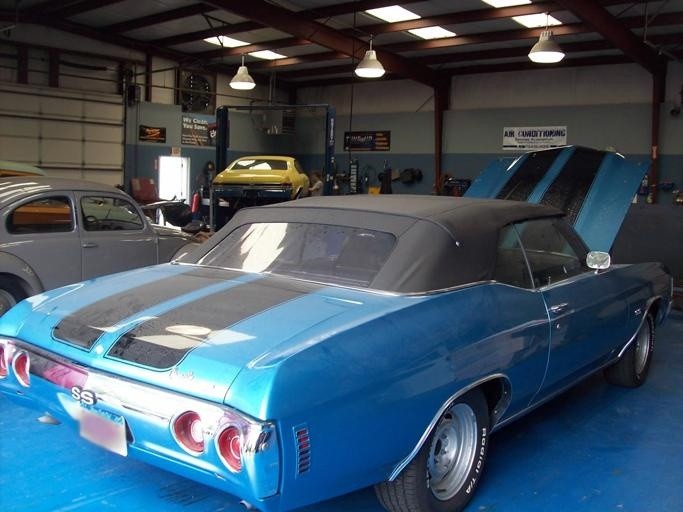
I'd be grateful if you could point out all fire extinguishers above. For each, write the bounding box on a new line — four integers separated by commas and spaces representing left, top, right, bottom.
191, 190, 200, 214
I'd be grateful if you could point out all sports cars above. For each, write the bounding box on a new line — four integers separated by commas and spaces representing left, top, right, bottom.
0, 143, 675, 512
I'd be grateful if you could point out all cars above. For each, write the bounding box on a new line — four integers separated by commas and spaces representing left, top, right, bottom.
0, 161, 131, 228
213, 154, 311, 201
0, 177, 199, 314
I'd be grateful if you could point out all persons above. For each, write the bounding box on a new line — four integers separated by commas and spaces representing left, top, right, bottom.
307, 170, 324, 196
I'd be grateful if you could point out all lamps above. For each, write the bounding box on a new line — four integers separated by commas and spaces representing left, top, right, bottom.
354, 35, 385, 78
528, 13, 566, 64
229, 54, 256, 90
